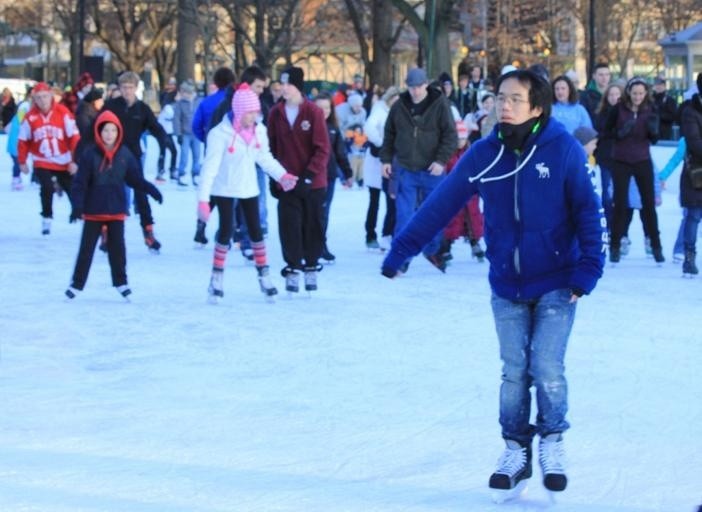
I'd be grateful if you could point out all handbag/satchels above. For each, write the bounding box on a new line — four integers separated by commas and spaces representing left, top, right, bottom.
685, 164, 702, 191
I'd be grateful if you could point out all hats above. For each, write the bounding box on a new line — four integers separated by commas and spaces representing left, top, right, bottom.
179, 78, 195, 96
117, 70, 141, 88
338, 72, 365, 110
438, 72, 454, 86
34, 79, 53, 95
83, 86, 104, 104
454, 118, 471, 141
404, 66, 428, 87
63, 71, 93, 94
230, 82, 263, 130
278, 67, 305, 92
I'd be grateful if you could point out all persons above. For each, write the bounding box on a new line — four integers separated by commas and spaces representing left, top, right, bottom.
380, 68, 608, 489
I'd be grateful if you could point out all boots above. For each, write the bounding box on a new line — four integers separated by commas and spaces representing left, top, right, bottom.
99, 224, 109, 252
255, 264, 278, 296
536, 429, 569, 492
194, 218, 209, 244
207, 266, 226, 299
487, 425, 542, 491
142, 222, 162, 249
682, 241, 699, 275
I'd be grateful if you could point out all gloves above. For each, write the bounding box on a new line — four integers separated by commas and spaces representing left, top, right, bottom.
140, 180, 164, 205
198, 200, 211, 225
295, 169, 316, 199
617, 119, 637, 139
68, 206, 84, 224
278, 171, 300, 193
647, 114, 660, 136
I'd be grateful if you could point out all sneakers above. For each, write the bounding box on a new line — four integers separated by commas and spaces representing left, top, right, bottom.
469, 238, 488, 258
341, 179, 364, 193
303, 263, 323, 291
42, 215, 53, 234
609, 236, 631, 264
427, 240, 454, 272
65, 281, 83, 298
671, 252, 685, 265
398, 261, 410, 273
10, 176, 23, 192
156, 168, 201, 187
365, 234, 392, 251
644, 236, 666, 264
318, 244, 336, 260
114, 283, 132, 299
280, 265, 301, 293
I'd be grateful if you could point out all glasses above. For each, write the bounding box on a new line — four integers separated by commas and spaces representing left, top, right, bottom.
494, 95, 530, 106
